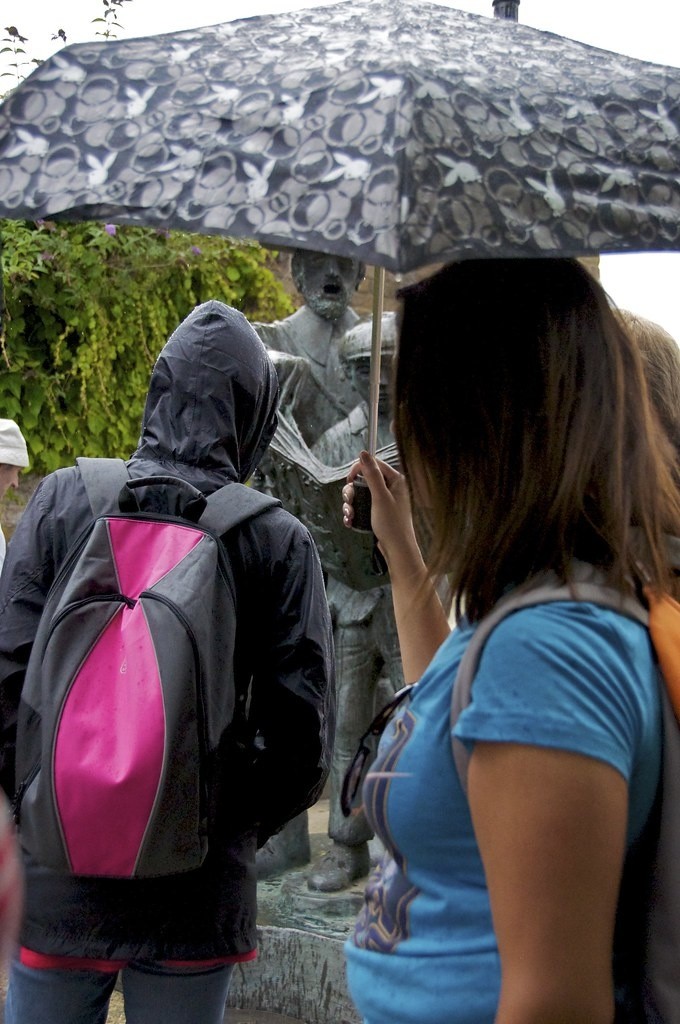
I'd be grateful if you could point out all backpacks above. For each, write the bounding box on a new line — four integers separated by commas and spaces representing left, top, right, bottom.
452, 546, 680, 1024
14, 456, 281, 880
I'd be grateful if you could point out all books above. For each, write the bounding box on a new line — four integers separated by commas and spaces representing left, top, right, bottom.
266, 406, 452, 589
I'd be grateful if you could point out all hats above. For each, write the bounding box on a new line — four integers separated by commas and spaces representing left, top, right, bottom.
0, 419, 29, 467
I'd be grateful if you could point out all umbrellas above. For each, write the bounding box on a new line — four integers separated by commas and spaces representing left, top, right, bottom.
0, 0, 680, 256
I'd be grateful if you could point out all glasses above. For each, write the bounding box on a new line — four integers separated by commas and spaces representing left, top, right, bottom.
340, 681, 418, 819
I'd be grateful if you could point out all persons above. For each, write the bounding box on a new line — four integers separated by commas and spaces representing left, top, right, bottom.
0, 301, 336, 1024
248, 248, 360, 881
342, 257, 662, 1024
0, 792, 25, 988
0, 419, 29, 568
614, 308, 680, 1024
309, 309, 401, 893
